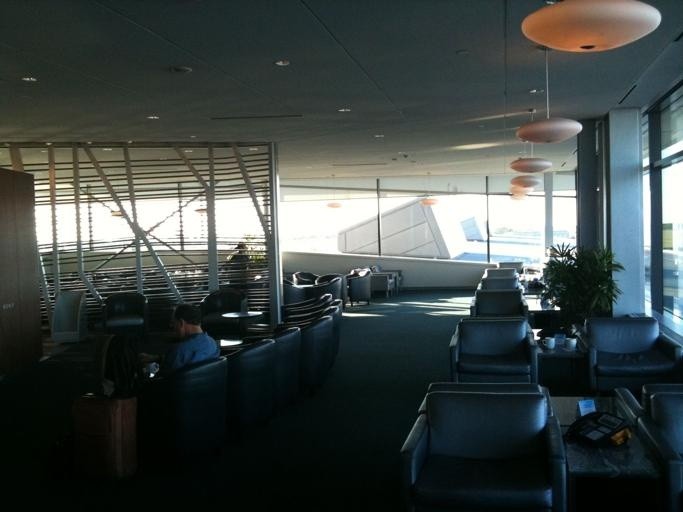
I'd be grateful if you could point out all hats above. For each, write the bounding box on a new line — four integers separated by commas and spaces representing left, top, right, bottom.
175, 304, 202, 325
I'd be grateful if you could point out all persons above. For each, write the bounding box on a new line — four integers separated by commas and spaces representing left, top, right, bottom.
130, 303, 222, 397
224, 242, 252, 290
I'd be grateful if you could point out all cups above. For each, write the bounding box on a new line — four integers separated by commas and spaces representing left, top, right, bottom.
542, 337, 555, 349
146, 362, 160, 374
565, 338, 577, 350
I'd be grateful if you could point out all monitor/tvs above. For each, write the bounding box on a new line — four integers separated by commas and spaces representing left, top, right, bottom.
220, 337, 245, 346
142, 359, 161, 378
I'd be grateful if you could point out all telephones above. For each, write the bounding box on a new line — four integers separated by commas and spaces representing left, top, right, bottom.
567, 412, 628, 447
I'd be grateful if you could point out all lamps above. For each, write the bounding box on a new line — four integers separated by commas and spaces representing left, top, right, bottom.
507, 0, 662, 193
